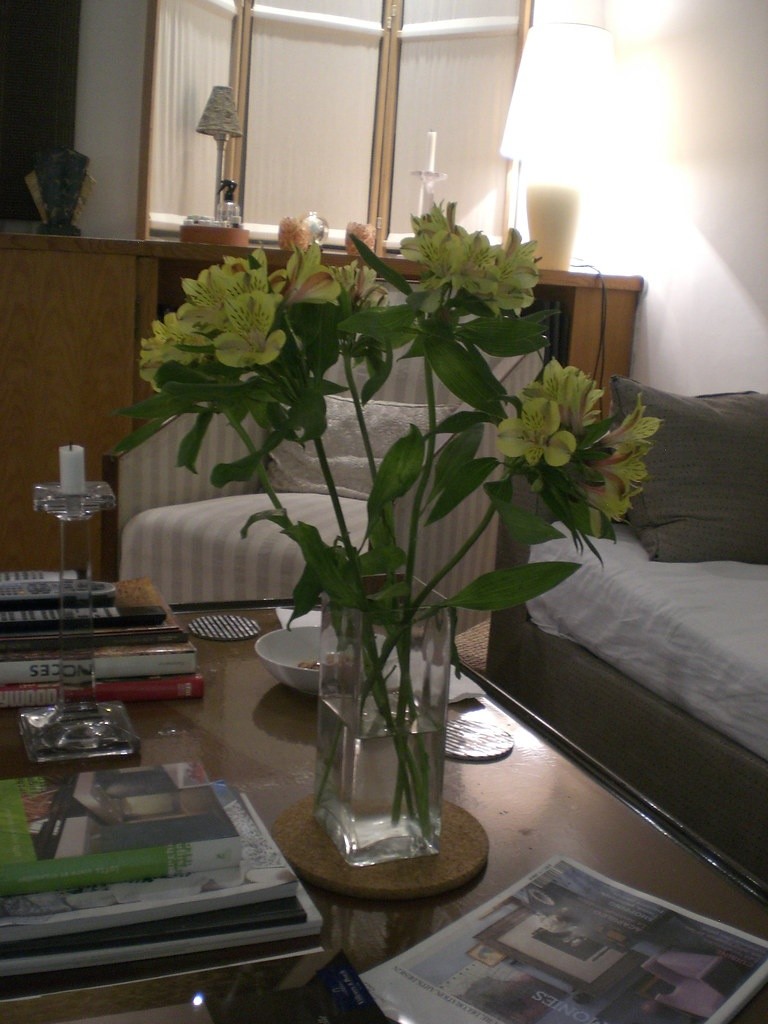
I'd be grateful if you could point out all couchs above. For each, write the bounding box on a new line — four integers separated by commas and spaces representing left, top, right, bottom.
482, 376, 766, 896
97, 388, 507, 658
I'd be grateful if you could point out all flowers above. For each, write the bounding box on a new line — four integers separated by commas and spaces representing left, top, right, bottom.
91, 193, 665, 846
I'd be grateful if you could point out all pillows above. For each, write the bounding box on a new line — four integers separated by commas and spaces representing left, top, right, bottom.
611, 376, 767, 566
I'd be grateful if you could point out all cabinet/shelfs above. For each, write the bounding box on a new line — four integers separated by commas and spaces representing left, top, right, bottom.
2, 234, 147, 583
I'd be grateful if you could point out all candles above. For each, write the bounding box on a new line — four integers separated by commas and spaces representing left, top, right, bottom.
410, 128, 448, 195
29, 438, 119, 525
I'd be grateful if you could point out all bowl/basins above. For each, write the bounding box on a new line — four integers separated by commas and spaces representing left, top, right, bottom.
254, 626, 320, 695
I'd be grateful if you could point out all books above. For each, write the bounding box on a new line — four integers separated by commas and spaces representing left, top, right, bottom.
0, 757, 388, 1023
359, 856, 767, 1023
0, 578, 204, 706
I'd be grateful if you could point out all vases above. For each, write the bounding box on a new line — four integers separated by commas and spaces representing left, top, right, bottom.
314, 571, 458, 868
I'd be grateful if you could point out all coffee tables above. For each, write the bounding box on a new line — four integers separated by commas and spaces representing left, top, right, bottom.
0, 598, 767, 1024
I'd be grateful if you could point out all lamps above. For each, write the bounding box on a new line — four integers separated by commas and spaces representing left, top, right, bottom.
497, 18, 624, 273
177, 84, 252, 247
